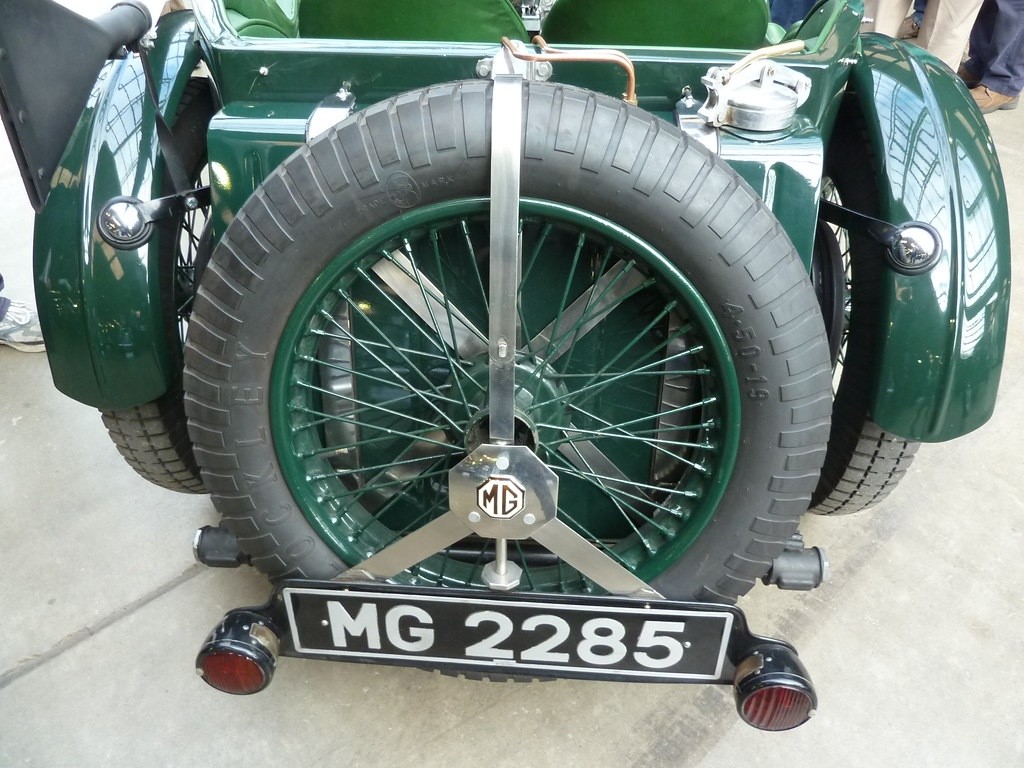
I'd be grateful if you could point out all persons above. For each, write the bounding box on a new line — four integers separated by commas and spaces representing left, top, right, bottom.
0, 271, 48, 356
767, 0, 1024, 116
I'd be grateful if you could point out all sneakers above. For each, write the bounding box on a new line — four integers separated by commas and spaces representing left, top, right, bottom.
0, 299, 46, 353
897, 17, 920, 38
955, 63, 981, 89
966, 84, 1019, 114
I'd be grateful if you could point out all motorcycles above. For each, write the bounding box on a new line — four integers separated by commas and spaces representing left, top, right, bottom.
32, 0, 1012, 735
